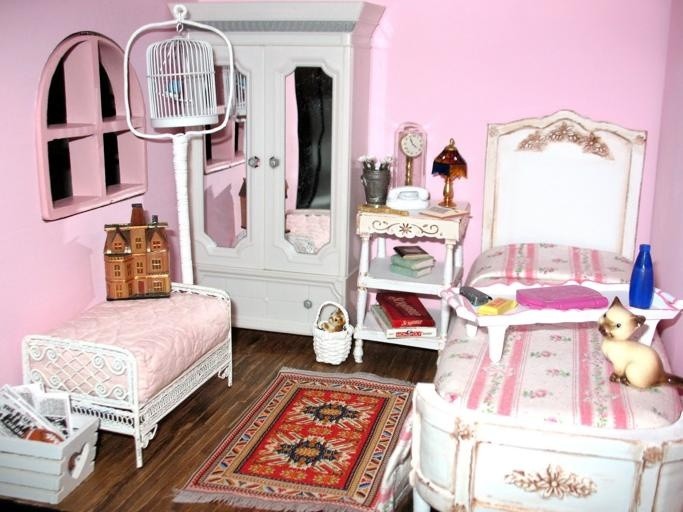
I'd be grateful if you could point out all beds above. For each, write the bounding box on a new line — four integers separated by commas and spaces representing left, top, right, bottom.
408, 109, 683, 511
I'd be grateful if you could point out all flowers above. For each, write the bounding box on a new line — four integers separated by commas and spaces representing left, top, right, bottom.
358, 151, 394, 171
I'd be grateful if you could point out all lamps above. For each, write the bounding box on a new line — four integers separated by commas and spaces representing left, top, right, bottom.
431, 138, 468, 208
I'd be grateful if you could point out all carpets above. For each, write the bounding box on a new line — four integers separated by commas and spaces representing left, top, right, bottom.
172, 366, 416, 512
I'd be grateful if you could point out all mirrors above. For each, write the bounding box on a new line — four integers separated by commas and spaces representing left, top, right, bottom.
200, 61, 246, 248
281, 66, 332, 254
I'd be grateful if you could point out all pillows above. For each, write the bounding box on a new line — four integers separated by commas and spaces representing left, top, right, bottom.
462, 242, 638, 285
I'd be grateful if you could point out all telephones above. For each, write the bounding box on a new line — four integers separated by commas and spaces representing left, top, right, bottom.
386, 186, 429, 211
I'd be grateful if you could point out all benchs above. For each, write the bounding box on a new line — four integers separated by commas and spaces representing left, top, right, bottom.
21, 281, 233, 469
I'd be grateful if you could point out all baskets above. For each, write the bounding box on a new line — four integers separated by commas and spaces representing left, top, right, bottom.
312, 301, 354, 365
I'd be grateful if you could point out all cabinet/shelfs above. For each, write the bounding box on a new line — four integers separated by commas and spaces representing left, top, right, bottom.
165, 0, 384, 337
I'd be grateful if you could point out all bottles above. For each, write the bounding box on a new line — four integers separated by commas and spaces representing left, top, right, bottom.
628, 244, 654, 310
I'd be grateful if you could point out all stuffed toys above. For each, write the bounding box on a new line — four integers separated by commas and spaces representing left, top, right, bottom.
319, 311, 345, 333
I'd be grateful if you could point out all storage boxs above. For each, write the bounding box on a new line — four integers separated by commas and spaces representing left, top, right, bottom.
0, 414, 100, 505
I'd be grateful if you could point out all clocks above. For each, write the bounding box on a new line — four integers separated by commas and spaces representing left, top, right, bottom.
392, 123, 427, 189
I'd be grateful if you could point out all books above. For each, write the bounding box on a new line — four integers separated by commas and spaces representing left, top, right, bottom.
391, 254, 434, 270
389, 264, 432, 278
393, 245, 427, 259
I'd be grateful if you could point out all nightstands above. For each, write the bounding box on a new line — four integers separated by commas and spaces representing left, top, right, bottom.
349, 204, 470, 367
